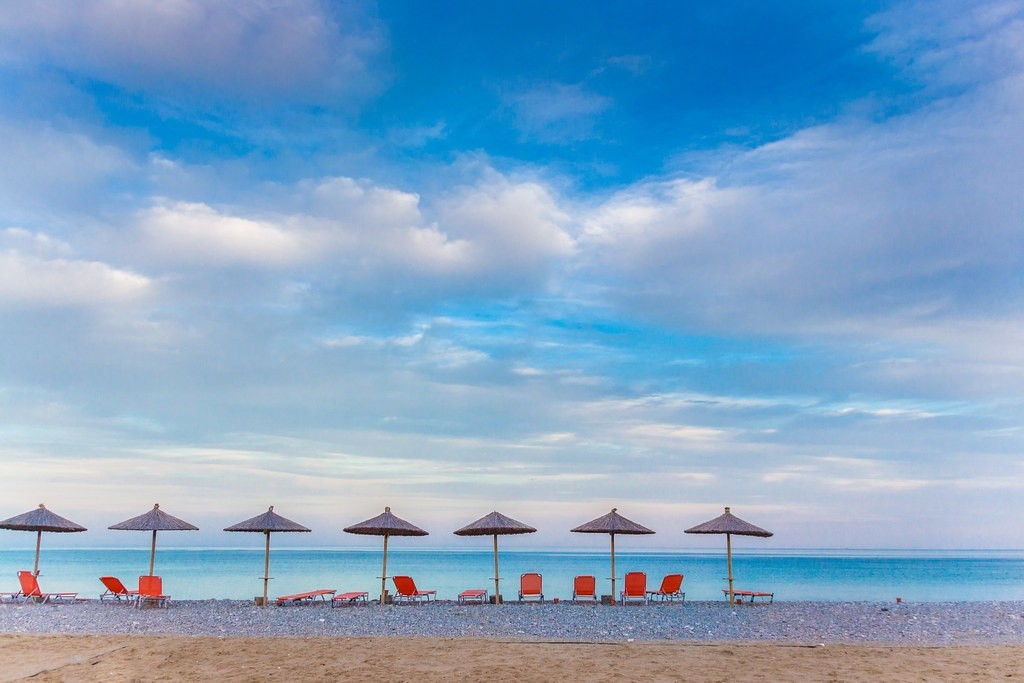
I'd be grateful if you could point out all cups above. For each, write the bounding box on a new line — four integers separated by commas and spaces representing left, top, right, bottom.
385, 590, 388, 595
554, 598, 559, 603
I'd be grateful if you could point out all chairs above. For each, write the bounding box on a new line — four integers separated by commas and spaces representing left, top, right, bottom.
392, 575, 438, 605
276, 587, 370, 609
620, 572, 649, 606
572, 575, 597, 605
0, 570, 79, 606
98, 575, 171, 610
458, 589, 489, 606
722, 589, 774, 604
518, 572, 545, 604
646, 574, 687, 605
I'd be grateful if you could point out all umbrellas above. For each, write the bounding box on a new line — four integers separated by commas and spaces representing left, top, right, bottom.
343, 507, 429, 604
684, 506, 775, 604
223, 506, 312, 605
570, 507, 656, 601
108, 502, 200, 576
453, 510, 537, 604
0, 503, 87, 578
